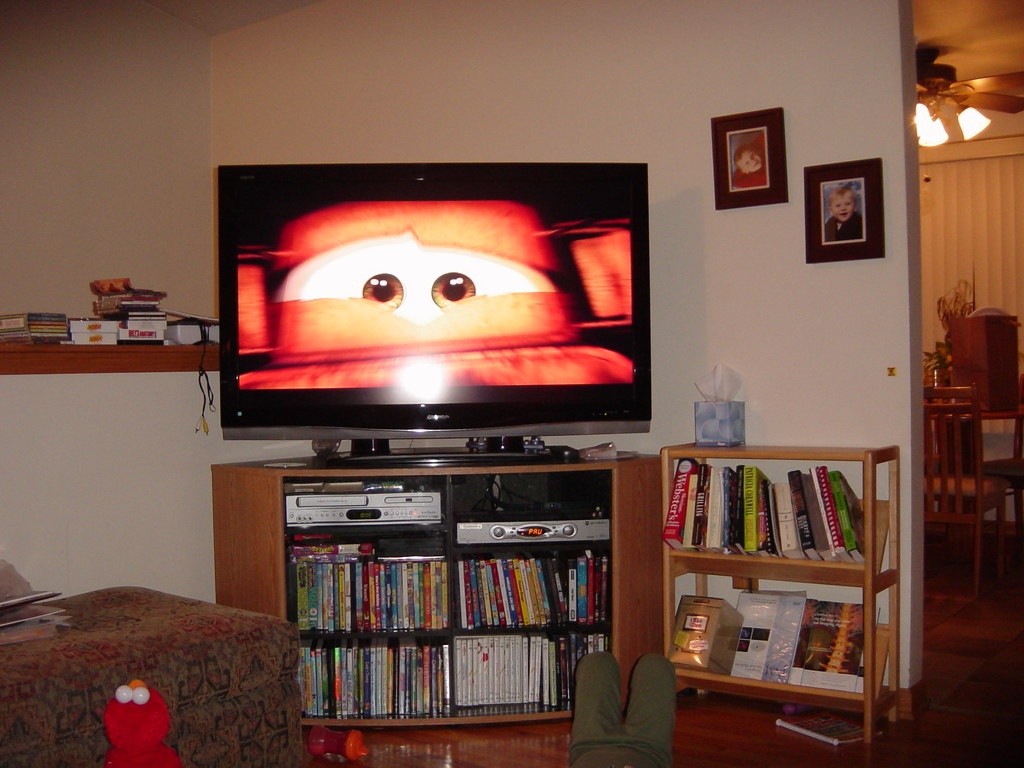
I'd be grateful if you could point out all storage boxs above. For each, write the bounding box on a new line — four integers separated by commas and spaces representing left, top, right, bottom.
666, 594, 745, 675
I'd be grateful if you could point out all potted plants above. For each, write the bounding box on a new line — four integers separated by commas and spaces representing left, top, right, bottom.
924, 341, 949, 387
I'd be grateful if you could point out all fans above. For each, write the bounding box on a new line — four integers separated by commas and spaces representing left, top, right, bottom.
916, 71, 1023, 114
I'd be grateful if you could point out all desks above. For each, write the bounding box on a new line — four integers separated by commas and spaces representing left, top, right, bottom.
926, 403, 1024, 420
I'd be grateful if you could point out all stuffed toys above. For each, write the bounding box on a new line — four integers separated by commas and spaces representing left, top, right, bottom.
104, 681, 183, 768
567, 651, 675, 768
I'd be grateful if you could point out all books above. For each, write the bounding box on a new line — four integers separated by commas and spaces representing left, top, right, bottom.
661, 459, 862, 564
0, 559, 65, 641
289, 542, 609, 716
775, 710, 884, 745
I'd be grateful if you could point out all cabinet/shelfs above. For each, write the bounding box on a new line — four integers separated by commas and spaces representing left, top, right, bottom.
211, 448, 660, 726
660, 446, 902, 745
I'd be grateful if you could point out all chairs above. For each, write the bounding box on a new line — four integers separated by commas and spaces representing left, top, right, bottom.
982, 373, 1024, 570
923, 387, 1010, 600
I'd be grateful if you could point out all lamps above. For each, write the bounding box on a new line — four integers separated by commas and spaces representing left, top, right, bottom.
916, 75, 991, 147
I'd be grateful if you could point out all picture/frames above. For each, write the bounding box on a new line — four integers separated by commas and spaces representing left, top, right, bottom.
804, 157, 885, 264
711, 107, 789, 210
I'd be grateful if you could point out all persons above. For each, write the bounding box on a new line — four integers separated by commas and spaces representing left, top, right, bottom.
825, 188, 862, 242
733, 146, 768, 188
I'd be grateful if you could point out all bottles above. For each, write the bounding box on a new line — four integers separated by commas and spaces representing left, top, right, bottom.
307, 725, 369, 760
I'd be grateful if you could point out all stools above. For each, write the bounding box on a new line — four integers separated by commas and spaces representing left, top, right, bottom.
0, 586, 300, 768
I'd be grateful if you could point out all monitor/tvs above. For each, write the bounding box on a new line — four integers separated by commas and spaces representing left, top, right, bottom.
218, 162, 653, 469
